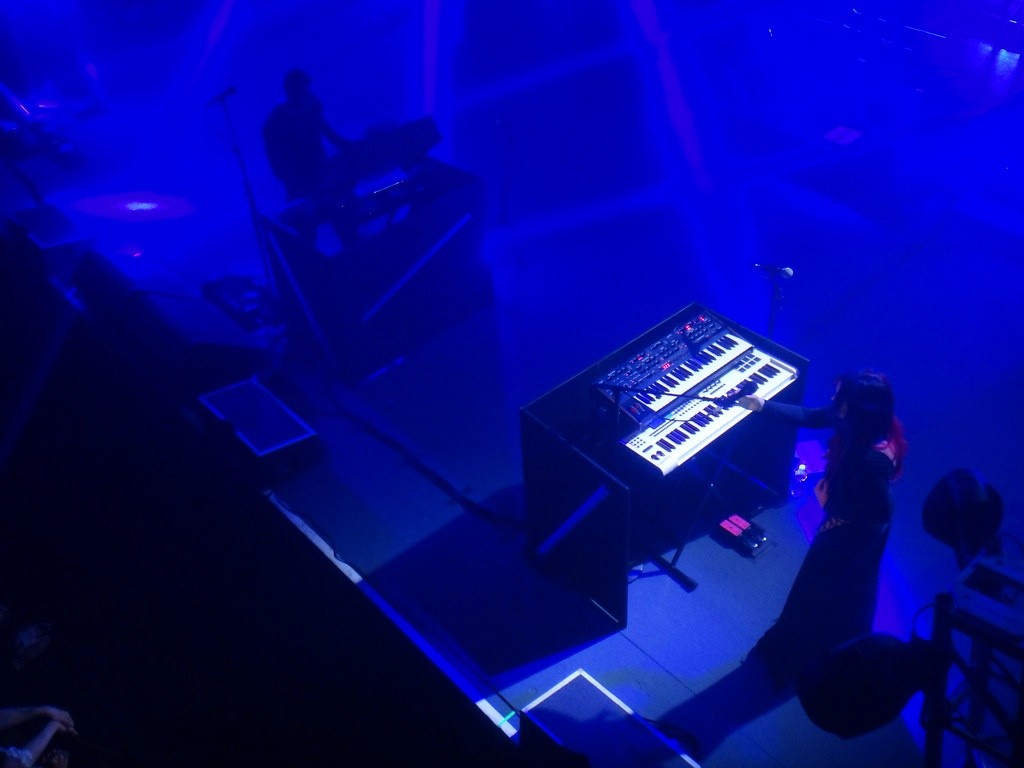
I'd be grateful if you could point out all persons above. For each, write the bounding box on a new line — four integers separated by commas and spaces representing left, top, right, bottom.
0, 703, 78, 768
738, 371, 905, 683
262, 70, 360, 250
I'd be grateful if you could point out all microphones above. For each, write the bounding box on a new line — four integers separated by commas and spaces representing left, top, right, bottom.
722, 381, 758, 410
211, 86, 237, 104
752, 263, 794, 279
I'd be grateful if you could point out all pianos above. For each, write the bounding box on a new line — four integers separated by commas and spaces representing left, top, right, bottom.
260, 113, 493, 366
518, 303, 813, 624
